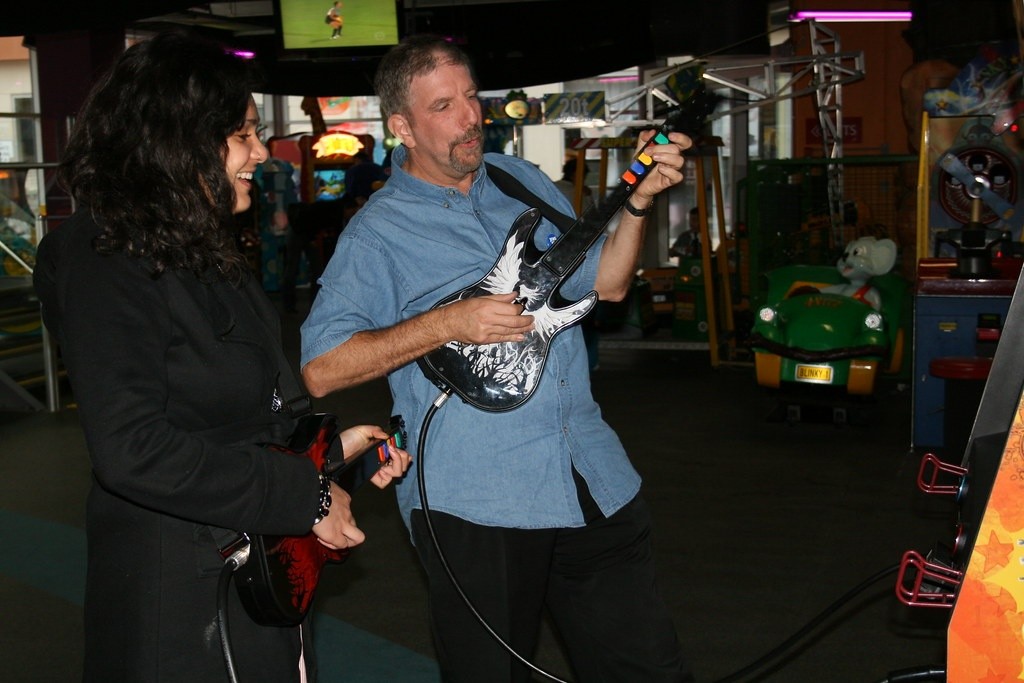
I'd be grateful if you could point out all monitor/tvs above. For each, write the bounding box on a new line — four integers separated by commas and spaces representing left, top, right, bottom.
272, 0, 408, 62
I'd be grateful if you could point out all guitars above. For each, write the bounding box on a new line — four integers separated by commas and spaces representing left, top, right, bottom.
416, 89, 721, 414
207, 411, 409, 627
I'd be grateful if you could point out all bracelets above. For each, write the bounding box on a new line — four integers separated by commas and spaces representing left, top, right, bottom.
622, 200, 657, 216
313, 467, 333, 526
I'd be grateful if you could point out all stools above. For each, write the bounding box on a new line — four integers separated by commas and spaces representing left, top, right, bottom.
928, 357, 994, 467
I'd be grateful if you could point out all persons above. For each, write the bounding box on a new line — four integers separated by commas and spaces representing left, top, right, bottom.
34, 38, 414, 682
347, 147, 394, 213
300, 32, 696, 681
324, 2, 344, 39
